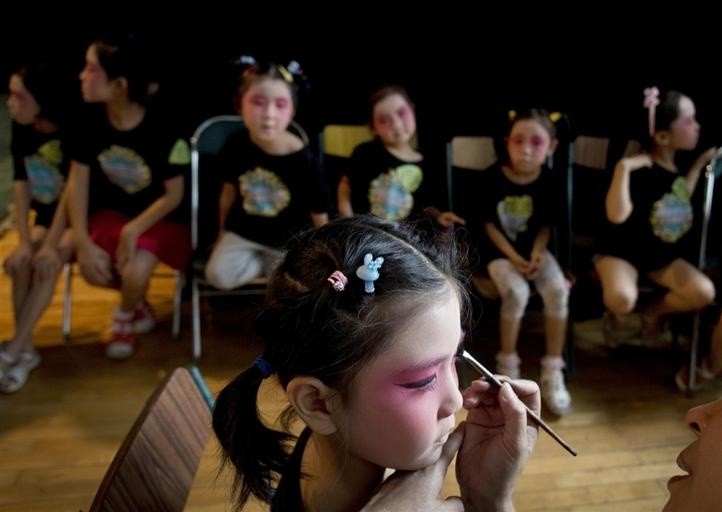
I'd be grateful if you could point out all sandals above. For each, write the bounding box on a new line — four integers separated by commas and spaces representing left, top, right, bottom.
0, 350, 16, 385
0, 347, 41, 392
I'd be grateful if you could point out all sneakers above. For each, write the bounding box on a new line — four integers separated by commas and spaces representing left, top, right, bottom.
106, 318, 134, 359
639, 307, 673, 349
675, 359, 716, 394
132, 303, 156, 341
603, 310, 627, 349
540, 370, 572, 414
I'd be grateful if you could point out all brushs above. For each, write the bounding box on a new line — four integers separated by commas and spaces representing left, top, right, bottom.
458, 344, 577, 457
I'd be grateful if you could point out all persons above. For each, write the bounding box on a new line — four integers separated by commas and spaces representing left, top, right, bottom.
466, 104, 570, 416
674, 312, 721, 391
212, 213, 463, 512
0, 57, 86, 395
359, 374, 722, 512
590, 87, 717, 346
201, 54, 330, 292
336, 80, 467, 227
65, 34, 190, 361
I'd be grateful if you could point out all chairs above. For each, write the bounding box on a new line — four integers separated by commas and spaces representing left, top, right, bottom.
64, 115, 722, 512
